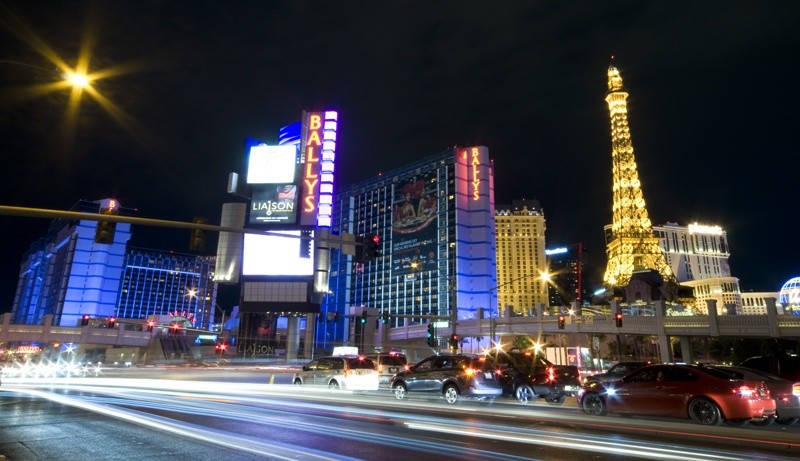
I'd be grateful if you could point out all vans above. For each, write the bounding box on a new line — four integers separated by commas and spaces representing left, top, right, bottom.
363, 353, 408, 384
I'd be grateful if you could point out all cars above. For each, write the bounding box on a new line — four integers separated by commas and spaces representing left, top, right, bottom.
578, 360, 800, 427
291, 355, 380, 391
389, 350, 582, 405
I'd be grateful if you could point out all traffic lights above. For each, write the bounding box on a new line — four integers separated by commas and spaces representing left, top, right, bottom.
333, 311, 338, 321
82, 314, 90, 326
452, 334, 460, 349
367, 233, 380, 259
146, 320, 154, 332
361, 310, 367, 323
382, 311, 389, 324
299, 232, 312, 258
107, 316, 116, 328
318, 312, 323, 322
172, 322, 181, 333
426, 324, 435, 347
615, 312, 623, 328
558, 314, 565, 328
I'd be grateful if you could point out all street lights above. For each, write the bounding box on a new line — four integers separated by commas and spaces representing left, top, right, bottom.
489, 272, 550, 350
206, 295, 225, 333
